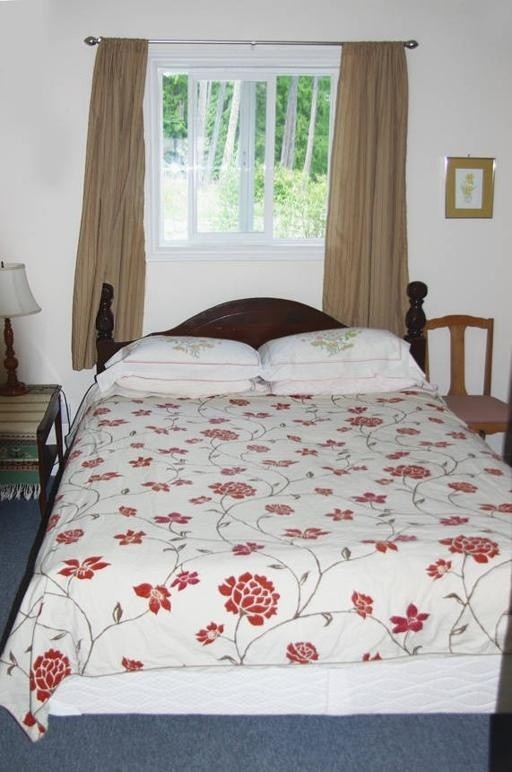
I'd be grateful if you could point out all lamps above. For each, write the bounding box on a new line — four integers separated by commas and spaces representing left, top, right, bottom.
0, 259, 42, 396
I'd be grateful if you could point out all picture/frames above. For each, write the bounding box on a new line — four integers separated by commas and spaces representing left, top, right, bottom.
442, 156, 497, 218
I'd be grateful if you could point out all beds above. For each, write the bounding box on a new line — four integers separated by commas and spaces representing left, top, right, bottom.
1, 274, 512, 745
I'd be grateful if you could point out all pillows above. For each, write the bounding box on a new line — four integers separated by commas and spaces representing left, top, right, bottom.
101, 376, 271, 404
97, 336, 265, 393
256, 328, 426, 382
259, 378, 439, 395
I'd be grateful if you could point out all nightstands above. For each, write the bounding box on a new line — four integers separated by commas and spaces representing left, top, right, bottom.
0, 384, 64, 521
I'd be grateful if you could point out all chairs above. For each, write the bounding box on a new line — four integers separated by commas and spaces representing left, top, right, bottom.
421, 313, 512, 463
